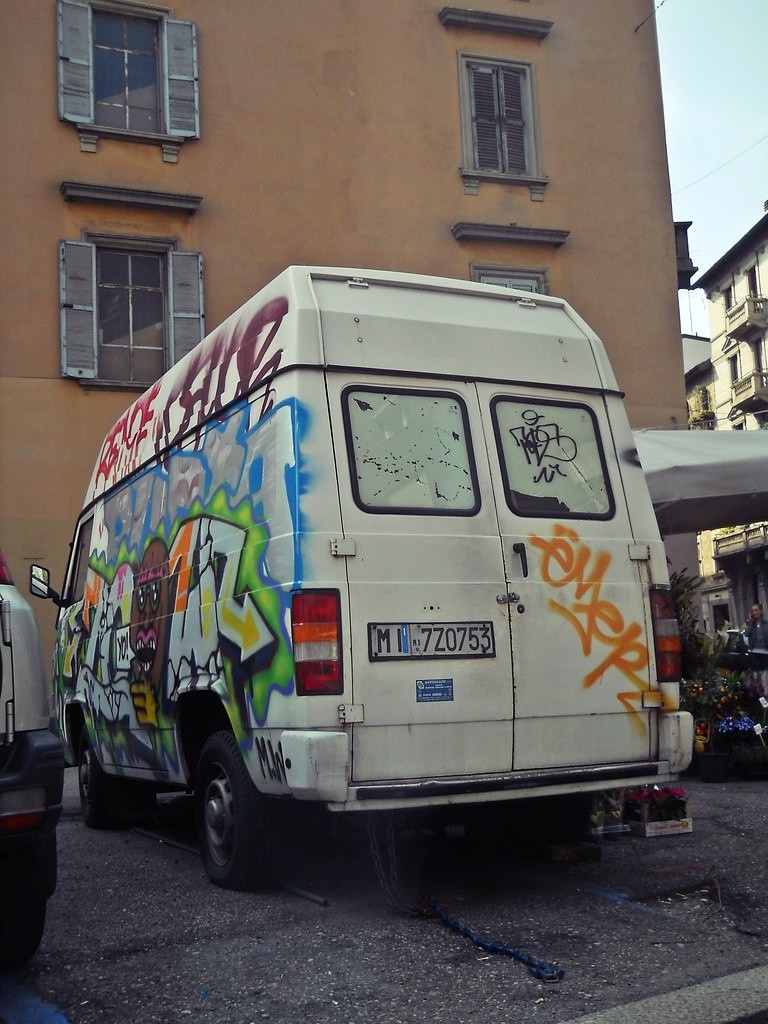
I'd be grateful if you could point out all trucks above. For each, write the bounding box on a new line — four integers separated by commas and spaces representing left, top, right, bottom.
28, 267, 698, 890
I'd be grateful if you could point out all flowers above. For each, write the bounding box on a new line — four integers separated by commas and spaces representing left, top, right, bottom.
581, 783, 690, 821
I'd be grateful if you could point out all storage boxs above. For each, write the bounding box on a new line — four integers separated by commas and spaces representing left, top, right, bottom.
623, 799, 693, 837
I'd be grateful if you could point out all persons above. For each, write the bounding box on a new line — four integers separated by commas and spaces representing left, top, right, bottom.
715, 616, 729, 653
745, 604, 768, 651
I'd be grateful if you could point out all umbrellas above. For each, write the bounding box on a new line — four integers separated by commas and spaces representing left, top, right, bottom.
565, 429, 768, 536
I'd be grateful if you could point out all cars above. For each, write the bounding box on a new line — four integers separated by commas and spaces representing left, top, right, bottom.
0, 555, 69, 973
